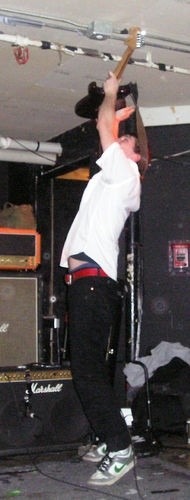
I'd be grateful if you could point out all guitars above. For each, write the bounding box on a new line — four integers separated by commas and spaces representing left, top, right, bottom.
74, 26, 147, 120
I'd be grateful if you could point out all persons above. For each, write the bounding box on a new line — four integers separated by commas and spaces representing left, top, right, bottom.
43, 72, 150, 487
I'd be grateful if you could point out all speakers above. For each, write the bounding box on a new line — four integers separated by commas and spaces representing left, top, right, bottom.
0, 271, 40, 369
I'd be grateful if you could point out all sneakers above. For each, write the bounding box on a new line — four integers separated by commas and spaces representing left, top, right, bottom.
83, 442, 111, 462
87, 444, 137, 486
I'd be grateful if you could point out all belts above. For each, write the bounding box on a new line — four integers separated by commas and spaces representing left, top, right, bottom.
63, 266, 108, 285
